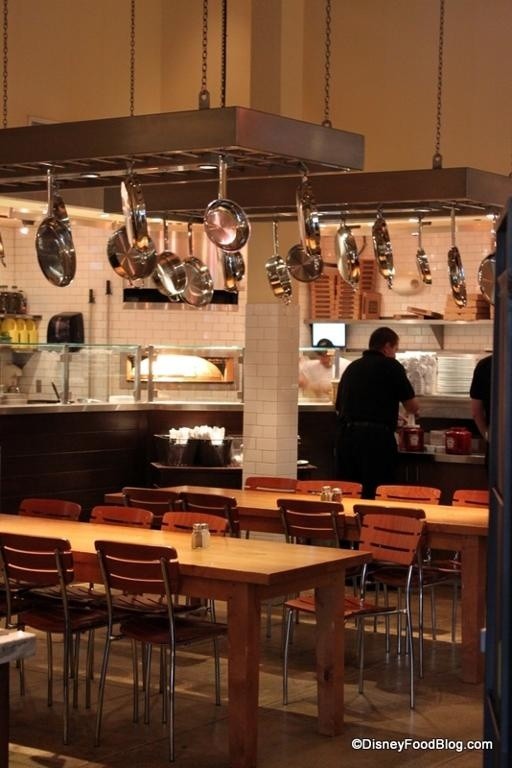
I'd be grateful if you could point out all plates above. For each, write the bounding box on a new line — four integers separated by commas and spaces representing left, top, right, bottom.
436, 355, 476, 393
296, 460, 310, 466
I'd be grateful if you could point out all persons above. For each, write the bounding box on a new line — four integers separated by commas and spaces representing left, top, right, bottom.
297, 339, 353, 400
469, 356, 493, 486
334, 327, 421, 499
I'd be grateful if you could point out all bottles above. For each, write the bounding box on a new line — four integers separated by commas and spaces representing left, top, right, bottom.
320, 485, 342, 503
0, 285, 27, 314
191, 523, 210, 548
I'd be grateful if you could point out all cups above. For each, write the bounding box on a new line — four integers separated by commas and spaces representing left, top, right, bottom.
427, 445, 445, 452
406, 355, 437, 396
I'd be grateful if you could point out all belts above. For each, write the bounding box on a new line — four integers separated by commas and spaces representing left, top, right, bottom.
347, 421, 389, 429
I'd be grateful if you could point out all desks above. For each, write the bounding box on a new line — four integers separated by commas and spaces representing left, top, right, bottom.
152, 461, 318, 491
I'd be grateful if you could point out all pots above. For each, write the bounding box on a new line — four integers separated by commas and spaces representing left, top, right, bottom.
36, 153, 497, 314
